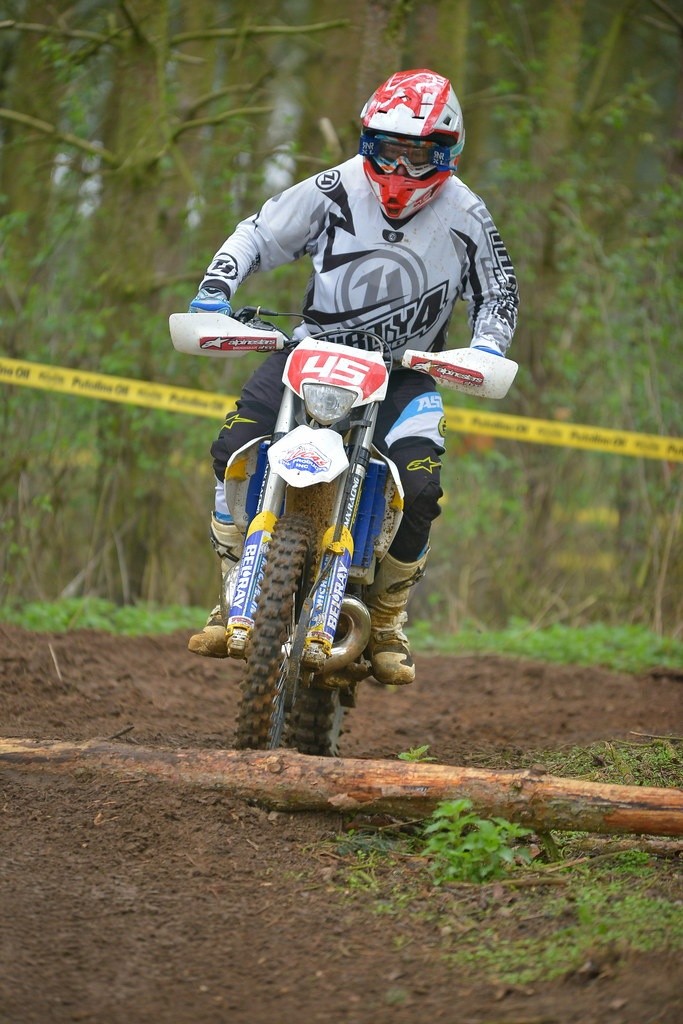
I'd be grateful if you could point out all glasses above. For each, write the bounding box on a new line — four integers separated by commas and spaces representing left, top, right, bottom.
358, 124, 466, 178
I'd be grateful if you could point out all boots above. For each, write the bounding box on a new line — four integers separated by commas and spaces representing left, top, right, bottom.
188, 511, 245, 659
354, 545, 432, 685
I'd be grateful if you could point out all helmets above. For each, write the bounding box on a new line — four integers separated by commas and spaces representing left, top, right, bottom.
360, 69, 464, 219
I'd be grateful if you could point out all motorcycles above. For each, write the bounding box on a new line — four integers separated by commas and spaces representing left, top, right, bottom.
169, 305, 520, 756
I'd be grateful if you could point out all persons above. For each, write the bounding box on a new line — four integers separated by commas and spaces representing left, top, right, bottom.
187, 69, 519, 686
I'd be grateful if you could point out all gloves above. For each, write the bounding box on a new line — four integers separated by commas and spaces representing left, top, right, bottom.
188, 287, 232, 316
